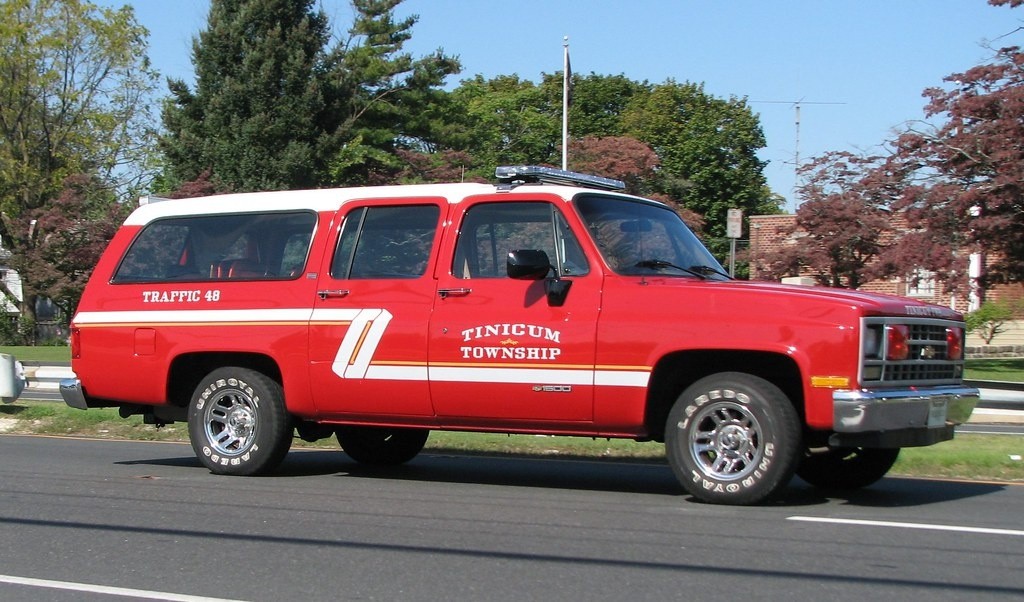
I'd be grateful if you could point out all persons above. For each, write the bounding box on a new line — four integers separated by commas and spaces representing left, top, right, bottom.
564, 227, 599, 270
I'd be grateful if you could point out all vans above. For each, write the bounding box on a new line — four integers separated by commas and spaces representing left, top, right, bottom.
59, 164, 981, 504
